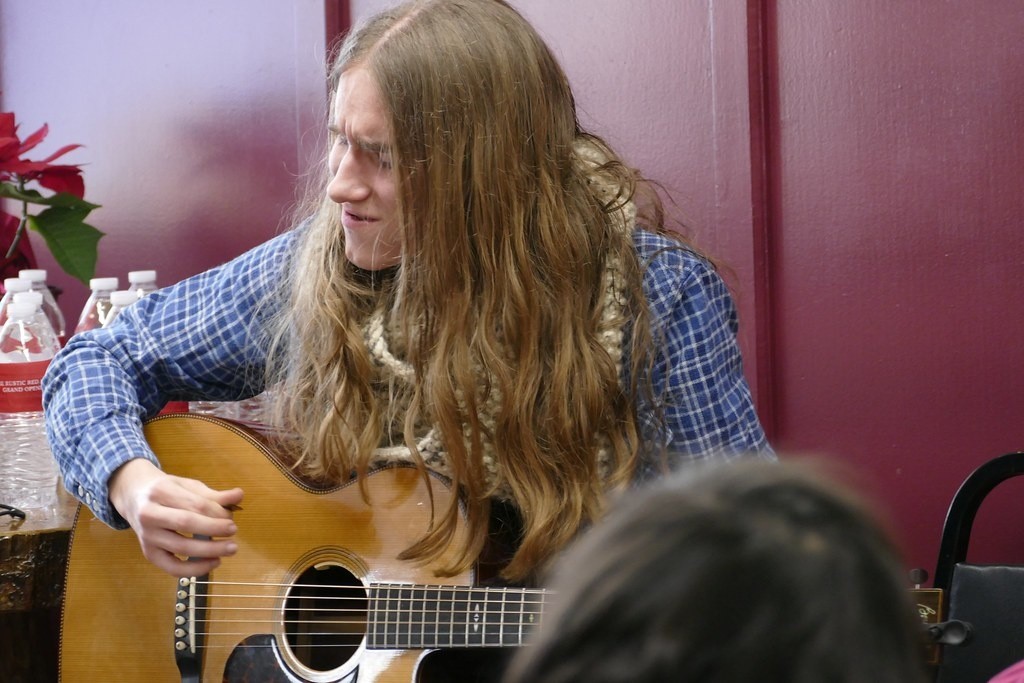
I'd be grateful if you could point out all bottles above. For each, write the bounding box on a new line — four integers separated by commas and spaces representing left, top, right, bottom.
19, 270, 67, 349
0, 278, 32, 335
0, 303, 56, 509
13, 292, 61, 484
128, 271, 160, 299
101, 290, 140, 327
74, 278, 118, 337
190, 391, 266, 423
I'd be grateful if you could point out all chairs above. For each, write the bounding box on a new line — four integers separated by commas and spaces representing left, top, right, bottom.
926, 451, 1024, 683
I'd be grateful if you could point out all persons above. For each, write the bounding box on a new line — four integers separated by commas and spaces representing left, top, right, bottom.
503, 456, 939, 683
41, 0, 779, 577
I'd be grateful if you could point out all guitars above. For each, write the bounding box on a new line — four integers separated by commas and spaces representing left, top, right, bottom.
56, 412, 948, 683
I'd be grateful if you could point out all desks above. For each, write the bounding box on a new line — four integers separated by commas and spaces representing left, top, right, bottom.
0, 412, 81, 683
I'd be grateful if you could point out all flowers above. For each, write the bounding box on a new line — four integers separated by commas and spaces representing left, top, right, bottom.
0, 111, 108, 294
